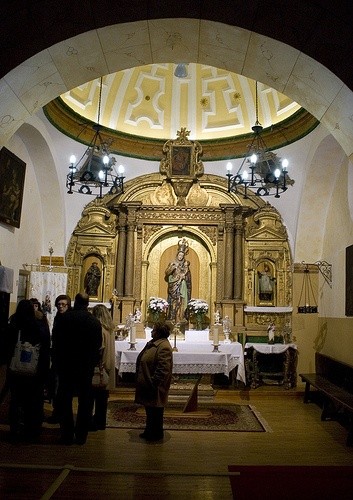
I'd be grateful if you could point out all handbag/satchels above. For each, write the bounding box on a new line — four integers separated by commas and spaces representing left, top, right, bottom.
92, 360, 109, 387
10, 342, 40, 373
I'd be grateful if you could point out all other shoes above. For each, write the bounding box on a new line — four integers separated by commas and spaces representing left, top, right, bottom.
139, 432, 162, 442
46, 415, 60, 424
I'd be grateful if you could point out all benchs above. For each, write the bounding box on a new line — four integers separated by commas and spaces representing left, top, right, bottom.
299, 351, 353, 448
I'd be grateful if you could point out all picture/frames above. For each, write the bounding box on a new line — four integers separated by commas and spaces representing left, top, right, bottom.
0, 146, 27, 228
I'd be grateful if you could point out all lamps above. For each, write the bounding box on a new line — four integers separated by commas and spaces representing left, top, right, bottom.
226, 79, 295, 199
296, 261, 333, 314
66, 76, 125, 200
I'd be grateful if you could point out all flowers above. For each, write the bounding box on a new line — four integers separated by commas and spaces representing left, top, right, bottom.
188, 298, 209, 315
148, 297, 170, 314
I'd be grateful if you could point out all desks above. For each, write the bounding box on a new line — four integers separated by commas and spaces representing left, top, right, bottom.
115, 340, 246, 389
244, 343, 298, 389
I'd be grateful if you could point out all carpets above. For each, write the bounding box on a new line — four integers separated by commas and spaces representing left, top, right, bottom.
92, 399, 273, 433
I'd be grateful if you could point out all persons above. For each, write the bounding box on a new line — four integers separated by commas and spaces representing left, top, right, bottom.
135, 322, 173, 442
0, 298, 50, 443
85, 262, 101, 296
165, 238, 192, 323
214, 310, 220, 323
223, 316, 231, 332
49, 293, 115, 445
258, 262, 274, 301
135, 308, 141, 317
267, 323, 275, 342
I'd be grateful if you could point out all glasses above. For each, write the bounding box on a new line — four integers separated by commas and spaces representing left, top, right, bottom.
57, 304, 68, 307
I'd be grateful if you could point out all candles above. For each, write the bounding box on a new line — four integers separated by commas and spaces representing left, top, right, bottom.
130, 327, 136, 343
213, 329, 219, 344
